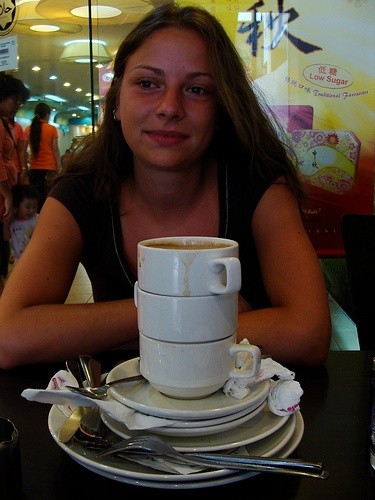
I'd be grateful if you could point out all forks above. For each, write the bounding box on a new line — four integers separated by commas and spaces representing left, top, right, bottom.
65, 353, 109, 450
102, 436, 329, 480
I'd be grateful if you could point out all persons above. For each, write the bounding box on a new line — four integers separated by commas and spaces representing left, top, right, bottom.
0, 71, 61, 298
0, 3, 331, 372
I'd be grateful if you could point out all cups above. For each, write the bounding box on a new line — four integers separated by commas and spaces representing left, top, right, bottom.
136, 235, 243, 297
137, 331, 261, 400
133, 281, 239, 343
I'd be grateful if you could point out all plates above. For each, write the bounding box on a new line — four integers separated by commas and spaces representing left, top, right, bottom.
98, 406, 289, 452
48, 404, 304, 490
106, 357, 272, 437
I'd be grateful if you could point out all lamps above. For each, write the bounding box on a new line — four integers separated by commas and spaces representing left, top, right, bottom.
60, 43, 113, 64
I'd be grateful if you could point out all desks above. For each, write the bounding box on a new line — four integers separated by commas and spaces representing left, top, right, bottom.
0, 350, 375, 500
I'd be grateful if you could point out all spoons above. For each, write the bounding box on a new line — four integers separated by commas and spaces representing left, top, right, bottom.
66, 375, 144, 400
